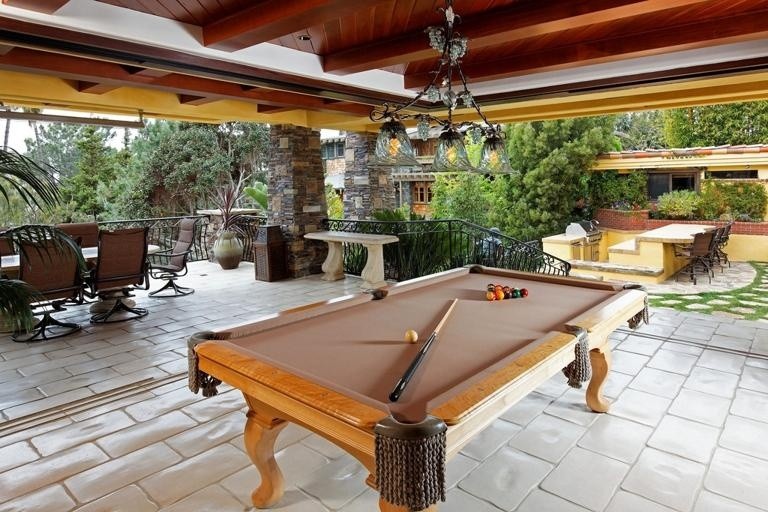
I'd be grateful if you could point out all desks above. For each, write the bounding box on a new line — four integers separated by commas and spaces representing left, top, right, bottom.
303, 230, 402, 296
188, 261, 657, 512
606, 223, 716, 281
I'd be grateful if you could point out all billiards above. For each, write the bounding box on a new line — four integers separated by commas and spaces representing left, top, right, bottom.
406, 330, 417, 343
487, 283, 527, 300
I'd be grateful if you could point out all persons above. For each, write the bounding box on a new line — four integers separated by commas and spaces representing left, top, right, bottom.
476, 227, 504, 266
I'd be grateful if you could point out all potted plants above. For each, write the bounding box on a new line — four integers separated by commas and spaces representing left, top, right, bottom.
205, 164, 258, 269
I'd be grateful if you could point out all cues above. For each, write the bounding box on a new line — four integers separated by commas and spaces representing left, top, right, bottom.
388, 298, 459, 400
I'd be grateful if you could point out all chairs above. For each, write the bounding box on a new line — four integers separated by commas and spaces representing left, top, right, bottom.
672, 219, 737, 286
1, 215, 206, 344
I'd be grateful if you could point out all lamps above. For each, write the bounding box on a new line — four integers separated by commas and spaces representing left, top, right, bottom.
366, 0, 523, 178
